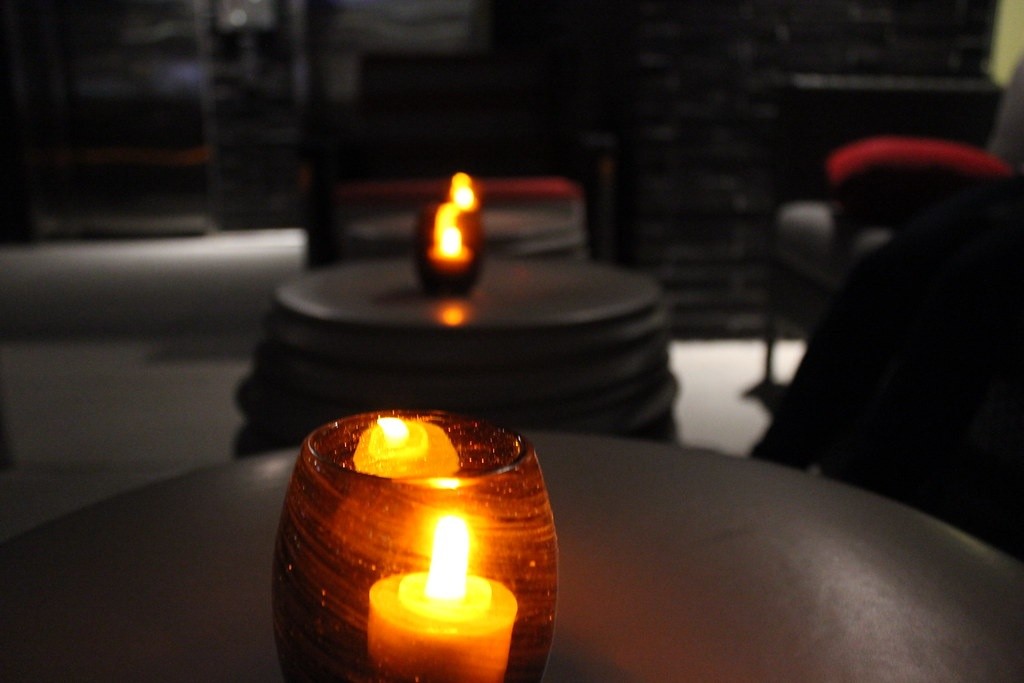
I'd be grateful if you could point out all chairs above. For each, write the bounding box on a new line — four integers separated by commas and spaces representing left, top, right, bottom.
759, 64, 1024, 403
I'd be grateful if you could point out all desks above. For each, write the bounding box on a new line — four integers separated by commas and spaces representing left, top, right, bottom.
0, 434, 1024, 683
232, 257, 684, 459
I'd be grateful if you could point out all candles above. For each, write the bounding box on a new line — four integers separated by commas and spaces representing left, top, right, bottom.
272, 411, 558, 683
